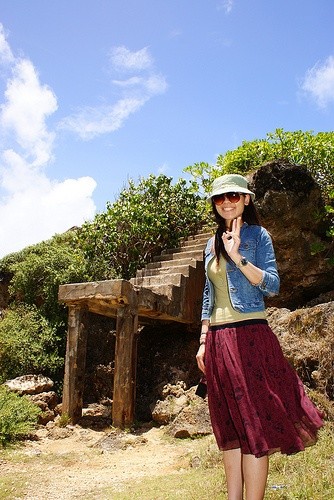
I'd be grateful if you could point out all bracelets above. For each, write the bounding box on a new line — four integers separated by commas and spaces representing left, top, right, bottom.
200, 332, 206, 345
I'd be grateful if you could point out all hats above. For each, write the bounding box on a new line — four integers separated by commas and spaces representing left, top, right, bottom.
206, 174, 255, 206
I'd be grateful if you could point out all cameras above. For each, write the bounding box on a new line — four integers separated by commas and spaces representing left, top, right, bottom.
195, 376, 208, 399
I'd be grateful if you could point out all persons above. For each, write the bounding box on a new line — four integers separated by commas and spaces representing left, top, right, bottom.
196, 174, 324, 500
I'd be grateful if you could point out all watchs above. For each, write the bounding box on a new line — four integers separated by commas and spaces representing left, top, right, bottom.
236, 257, 249, 269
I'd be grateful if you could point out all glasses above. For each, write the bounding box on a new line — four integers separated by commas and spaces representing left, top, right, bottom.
212, 192, 245, 206
